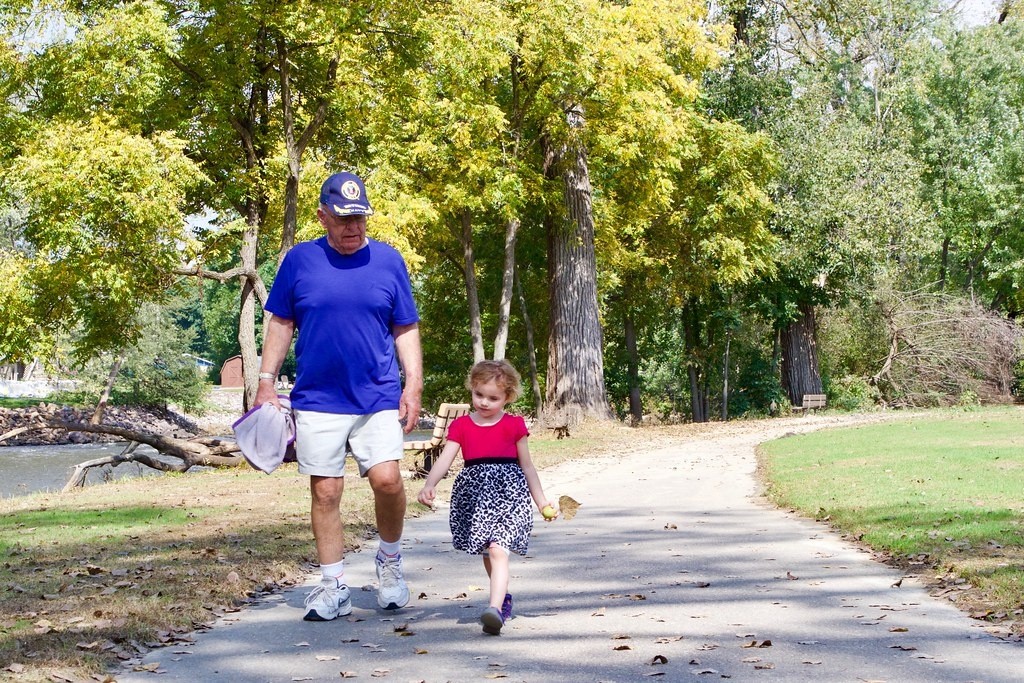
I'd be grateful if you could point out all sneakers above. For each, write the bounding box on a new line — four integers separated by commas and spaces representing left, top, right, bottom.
375, 550, 410, 610
502, 594, 512, 621
303, 576, 352, 620
481, 607, 505, 635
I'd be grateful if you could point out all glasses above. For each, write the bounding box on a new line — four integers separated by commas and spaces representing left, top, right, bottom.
323, 208, 369, 225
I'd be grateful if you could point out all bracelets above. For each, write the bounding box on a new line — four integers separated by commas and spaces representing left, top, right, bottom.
258, 372, 276, 381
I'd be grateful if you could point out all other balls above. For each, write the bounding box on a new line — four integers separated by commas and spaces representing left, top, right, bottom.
542, 506, 557, 519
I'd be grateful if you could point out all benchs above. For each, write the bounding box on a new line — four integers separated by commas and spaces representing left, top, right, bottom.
402, 402, 470, 479
792, 394, 827, 418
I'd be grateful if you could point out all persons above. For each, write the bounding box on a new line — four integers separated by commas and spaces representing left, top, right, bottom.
414, 359, 558, 636
252, 172, 426, 621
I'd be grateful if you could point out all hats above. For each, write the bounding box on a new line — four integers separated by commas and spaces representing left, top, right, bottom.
320, 172, 374, 216
